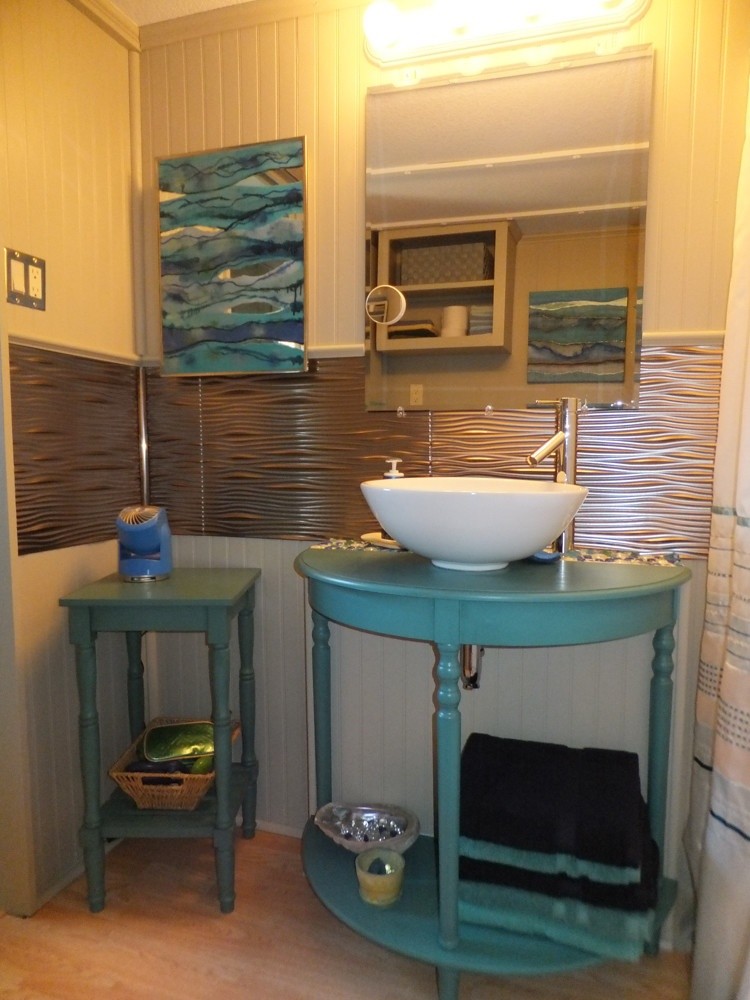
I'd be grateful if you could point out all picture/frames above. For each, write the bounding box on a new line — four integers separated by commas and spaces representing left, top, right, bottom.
155, 134, 308, 377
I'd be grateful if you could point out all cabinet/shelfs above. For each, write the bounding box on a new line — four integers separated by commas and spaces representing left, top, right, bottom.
297, 546, 692, 999
58, 567, 263, 915
375, 219, 524, 355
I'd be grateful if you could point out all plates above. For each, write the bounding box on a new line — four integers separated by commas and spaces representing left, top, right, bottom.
360, 532, 399, 550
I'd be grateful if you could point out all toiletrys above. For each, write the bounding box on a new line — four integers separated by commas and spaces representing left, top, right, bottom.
381, 457, 405, 542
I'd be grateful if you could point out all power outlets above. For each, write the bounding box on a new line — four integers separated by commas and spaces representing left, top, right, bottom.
409, 384, 423, 406
29, 264, 42, 300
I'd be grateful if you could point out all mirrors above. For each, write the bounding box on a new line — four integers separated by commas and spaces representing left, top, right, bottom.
366, 285, 407, 325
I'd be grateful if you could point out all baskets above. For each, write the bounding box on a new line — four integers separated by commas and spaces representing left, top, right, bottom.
109, 718, 242, 811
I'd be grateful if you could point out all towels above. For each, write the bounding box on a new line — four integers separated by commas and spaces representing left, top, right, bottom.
457, 730, 658, 967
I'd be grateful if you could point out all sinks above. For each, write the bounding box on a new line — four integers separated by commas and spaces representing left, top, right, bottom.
359, 476, 590, 572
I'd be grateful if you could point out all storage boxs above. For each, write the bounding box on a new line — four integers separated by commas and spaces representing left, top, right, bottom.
400, 242, 495, 285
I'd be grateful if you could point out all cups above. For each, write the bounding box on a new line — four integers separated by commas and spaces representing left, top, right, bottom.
355, 847, 405, 905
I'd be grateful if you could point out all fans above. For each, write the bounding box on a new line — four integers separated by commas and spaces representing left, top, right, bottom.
115, 505, 173, 583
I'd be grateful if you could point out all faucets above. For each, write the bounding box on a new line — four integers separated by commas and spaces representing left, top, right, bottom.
525, 396, 578, 554
